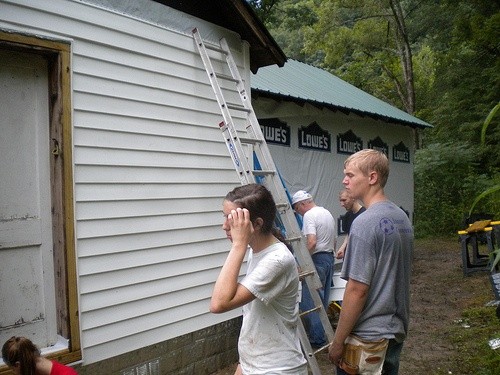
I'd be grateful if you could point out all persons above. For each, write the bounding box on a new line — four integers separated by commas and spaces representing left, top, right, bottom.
1, 336, 79, 375
210, 184, 308, 375
292, 149, 414, 375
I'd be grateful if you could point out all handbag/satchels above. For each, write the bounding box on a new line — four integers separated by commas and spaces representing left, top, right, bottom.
337, 334, 389, 375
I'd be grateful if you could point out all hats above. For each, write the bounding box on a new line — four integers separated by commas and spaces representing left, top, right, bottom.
292, 190, 312, 205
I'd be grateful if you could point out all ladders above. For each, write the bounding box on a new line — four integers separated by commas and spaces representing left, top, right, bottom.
192, 27, 334, 375
